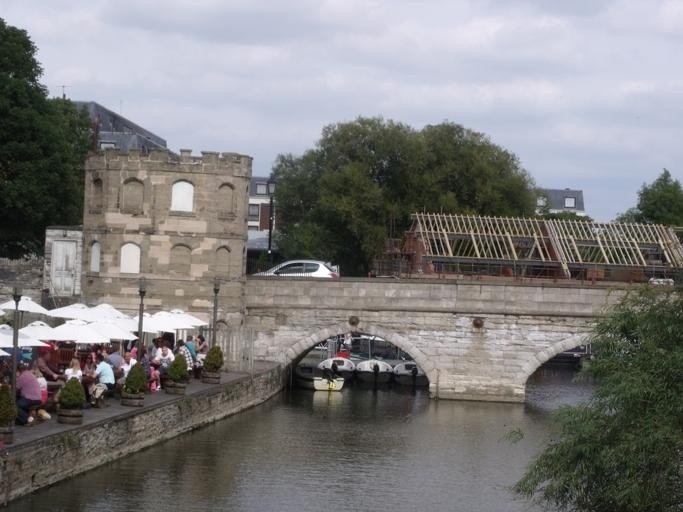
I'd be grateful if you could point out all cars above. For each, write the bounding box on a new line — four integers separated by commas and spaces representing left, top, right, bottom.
251, 260, 340, 278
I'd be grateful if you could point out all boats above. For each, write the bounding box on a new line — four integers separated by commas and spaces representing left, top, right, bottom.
294, 336, 427, 390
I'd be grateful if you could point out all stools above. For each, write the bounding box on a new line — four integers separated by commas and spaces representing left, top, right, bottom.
92, 390, 112, 408
24, 404, 41, 427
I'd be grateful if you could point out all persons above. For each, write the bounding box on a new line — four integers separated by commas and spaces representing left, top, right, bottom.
323, 365, 334, 378
332, 360, 337, 373
0, 335, 210, 430
344, 333, 354, 353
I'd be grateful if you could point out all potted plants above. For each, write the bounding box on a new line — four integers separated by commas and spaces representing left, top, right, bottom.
202, 346, 224, 384
0, 383, 17, 444
58, 377, 86, 425
121, 362, 147, 407
166, 354, 187, 395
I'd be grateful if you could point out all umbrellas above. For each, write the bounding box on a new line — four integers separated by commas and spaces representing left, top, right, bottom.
0, 295, 209, 359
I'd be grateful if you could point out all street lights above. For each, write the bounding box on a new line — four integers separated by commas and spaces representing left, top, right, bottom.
9, 262, 25, 430
138, 275, 148, 365
211, 275, 221, 348
265, 174, 278, 261
